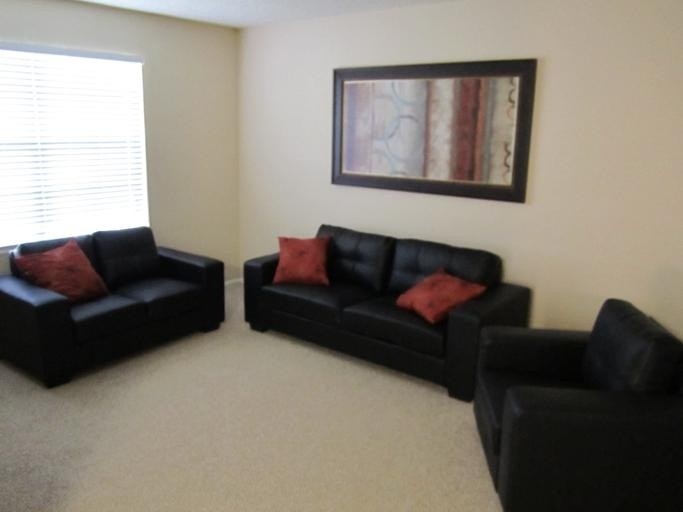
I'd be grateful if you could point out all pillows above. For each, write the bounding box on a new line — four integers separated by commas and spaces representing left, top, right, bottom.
273, 233, 331, 288
11, 238, 107, 310
395, 270, 487, 323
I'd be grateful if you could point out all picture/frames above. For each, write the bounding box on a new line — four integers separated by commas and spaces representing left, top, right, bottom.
329, 58, 538, 204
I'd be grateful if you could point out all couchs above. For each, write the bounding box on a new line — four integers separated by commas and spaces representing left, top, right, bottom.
0, 226, 225, 391
243, 224, 531, 403
471, 298, 683, 512
